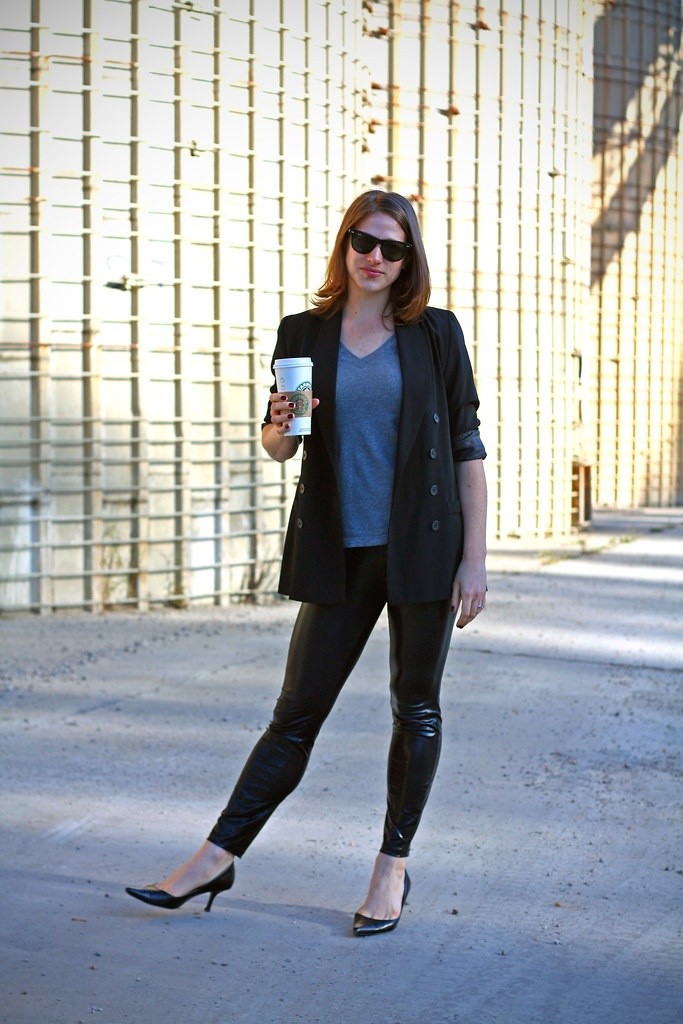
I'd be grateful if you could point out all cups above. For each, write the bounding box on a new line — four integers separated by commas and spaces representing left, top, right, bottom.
272, 357, 313, 436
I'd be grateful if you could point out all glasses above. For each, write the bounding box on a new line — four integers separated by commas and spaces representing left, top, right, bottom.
349, 228, 412, 263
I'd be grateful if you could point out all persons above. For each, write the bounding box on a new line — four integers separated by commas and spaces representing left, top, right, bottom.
124, 190, 491, 936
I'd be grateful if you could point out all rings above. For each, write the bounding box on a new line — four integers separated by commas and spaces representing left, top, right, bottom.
478, 605, 484, 609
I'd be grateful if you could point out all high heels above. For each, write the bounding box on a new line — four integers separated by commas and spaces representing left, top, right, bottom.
354, 868, 411, 935
125, 862, 235, 911
